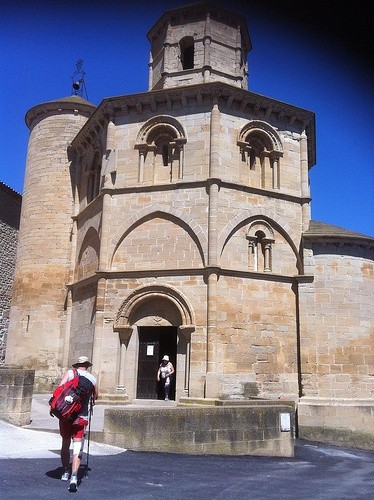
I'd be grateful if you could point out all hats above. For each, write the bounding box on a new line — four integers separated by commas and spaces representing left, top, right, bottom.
72, 356, 93, 368
162, 355, 169, 361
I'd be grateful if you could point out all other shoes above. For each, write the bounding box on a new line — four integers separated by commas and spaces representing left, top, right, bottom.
165, 397, 168, 401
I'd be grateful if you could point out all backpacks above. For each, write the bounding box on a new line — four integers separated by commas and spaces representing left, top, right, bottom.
49, 369, 94, 425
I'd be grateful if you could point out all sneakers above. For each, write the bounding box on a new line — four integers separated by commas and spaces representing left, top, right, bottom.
68, 476, 77, 493
61, 473, 70, 480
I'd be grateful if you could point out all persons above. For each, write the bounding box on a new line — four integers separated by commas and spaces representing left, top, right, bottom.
52, 356, 98, 492
157, 355, 174, 401
